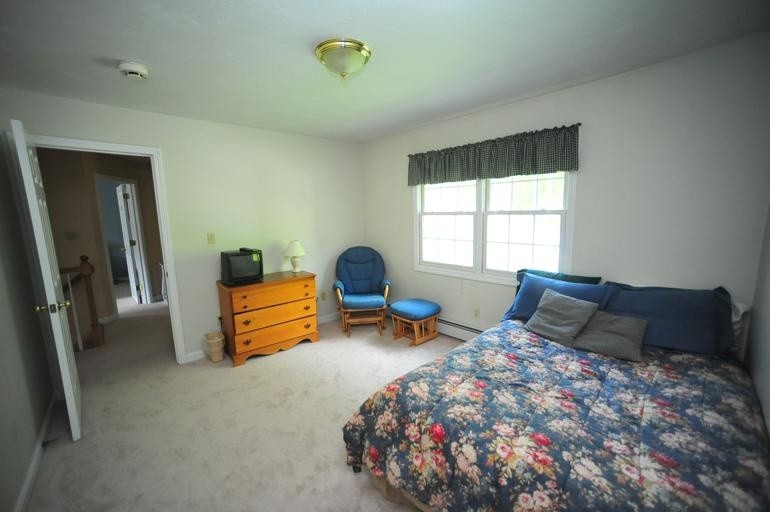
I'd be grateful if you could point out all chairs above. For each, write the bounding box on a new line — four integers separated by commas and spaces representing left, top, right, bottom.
333, 245, 392, 338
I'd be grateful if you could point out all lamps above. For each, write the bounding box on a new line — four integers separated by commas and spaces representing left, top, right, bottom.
117, 60, 148, 82
315, 37, 372, 79
284, 240, 306, 273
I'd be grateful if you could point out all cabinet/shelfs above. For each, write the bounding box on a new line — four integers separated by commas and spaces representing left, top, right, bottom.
216, 270, 320, 367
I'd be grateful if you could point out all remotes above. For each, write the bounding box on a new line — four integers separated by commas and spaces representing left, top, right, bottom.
241, 247, 254, 252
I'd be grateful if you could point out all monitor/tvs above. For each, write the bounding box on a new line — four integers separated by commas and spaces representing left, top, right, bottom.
220, 250, 264, 286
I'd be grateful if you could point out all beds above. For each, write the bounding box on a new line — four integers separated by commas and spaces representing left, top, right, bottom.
342, 305, 770, 512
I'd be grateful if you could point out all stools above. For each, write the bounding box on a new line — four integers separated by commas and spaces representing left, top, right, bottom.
389, 297, 441, 346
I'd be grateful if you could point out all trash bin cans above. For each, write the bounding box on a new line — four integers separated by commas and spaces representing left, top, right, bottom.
203, 332, 225, 362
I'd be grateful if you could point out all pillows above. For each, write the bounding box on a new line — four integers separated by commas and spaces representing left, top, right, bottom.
503, 268, 754, 362
